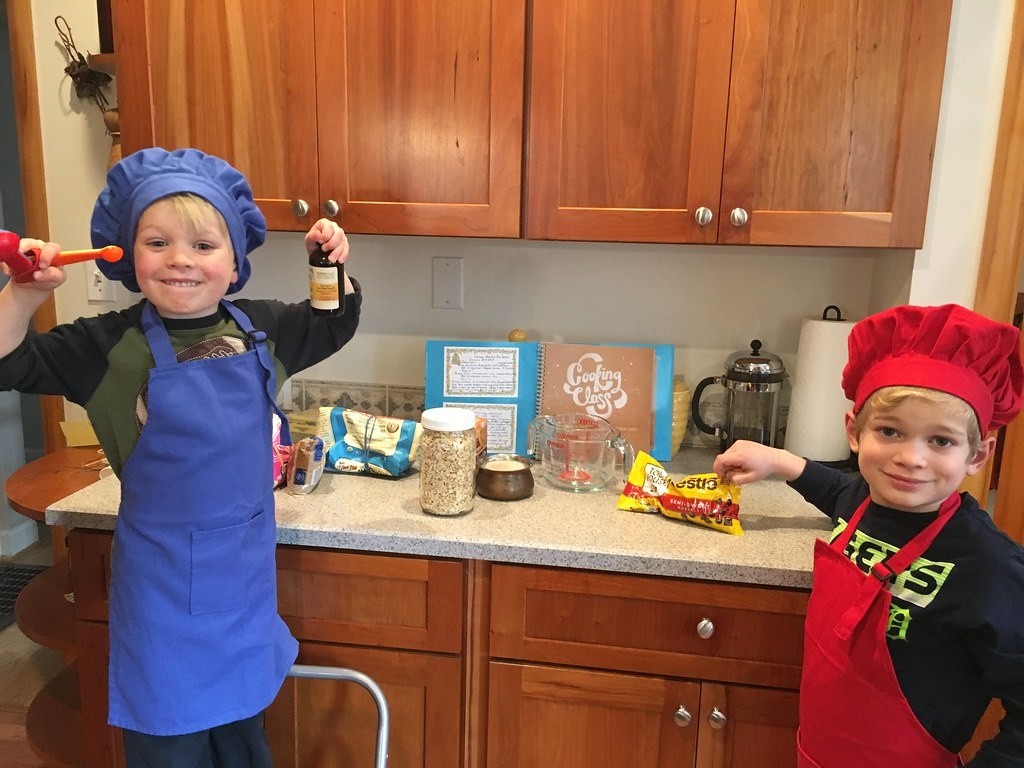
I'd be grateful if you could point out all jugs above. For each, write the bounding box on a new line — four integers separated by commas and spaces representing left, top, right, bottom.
691, 338, 791, 454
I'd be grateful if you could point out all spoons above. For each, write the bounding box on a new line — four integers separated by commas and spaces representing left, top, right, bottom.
47, 245, 124, 267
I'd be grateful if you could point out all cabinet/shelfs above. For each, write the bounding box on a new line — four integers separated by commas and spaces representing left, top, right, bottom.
66, 525, 468, 768
5, 445, 109, 768
522, 0, 953, 248
468, 558, 814, 768
110, 0, 528, 240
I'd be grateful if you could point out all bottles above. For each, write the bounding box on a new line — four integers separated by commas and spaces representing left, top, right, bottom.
307, 240, 346, 320
416, 405, 478, 520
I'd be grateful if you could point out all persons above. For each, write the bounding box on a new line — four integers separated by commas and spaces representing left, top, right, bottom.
712, 304, 1023, 767
0, 148, 361, 768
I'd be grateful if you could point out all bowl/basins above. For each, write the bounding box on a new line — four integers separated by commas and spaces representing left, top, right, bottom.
476, 451, 534, 502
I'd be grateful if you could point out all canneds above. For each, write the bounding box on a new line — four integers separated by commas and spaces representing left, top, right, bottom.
417, 407, 477, 516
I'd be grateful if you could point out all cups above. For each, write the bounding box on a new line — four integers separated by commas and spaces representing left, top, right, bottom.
672, 379, 691, 458
525, 409, 634, 495
